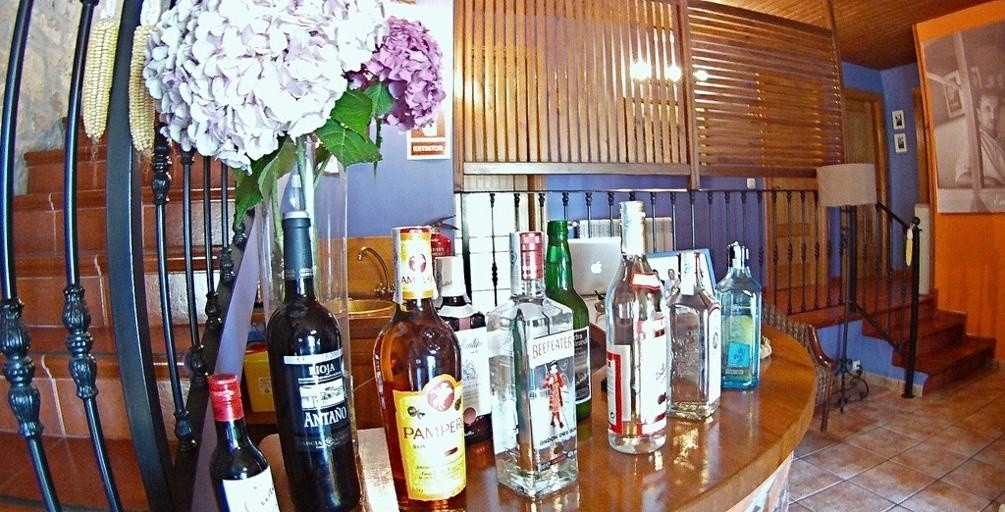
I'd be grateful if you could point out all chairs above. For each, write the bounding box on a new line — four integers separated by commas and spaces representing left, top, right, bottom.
761, 297, 838, 431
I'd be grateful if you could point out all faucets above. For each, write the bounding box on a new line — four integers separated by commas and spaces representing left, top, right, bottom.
357, 245, 394, 298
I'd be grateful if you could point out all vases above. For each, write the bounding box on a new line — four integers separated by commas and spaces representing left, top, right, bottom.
254, 131, 364, 458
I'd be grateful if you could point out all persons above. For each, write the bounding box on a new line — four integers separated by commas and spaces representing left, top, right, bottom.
955, 88, 1005, 187
664, 269, 679, 292
652, 270, 664, 287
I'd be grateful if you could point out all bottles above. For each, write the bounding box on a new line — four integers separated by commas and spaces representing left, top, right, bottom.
605, 200, 674, 457
715, 241, 762, 396
375, 225, 472, 510
484, 228, 583, 499
668, 248, 724, 424
265, 210, 363, 511
428, 251, 496, 448
202, 369, 281, 512
539, 217, 592, 424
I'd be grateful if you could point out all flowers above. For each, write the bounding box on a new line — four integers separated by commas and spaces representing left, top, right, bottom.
142, 0, 447, 301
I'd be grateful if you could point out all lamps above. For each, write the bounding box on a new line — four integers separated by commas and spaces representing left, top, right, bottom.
814, 162, 878, 414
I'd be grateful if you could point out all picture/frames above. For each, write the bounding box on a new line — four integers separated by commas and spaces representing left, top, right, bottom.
892, 109, 905, 130
894, 133, 908, 154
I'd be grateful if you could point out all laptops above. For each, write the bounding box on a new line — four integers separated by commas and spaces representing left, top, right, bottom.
567, 238, 620, 295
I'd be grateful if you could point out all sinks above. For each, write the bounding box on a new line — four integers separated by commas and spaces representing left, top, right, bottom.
328, 299, 394, 315
